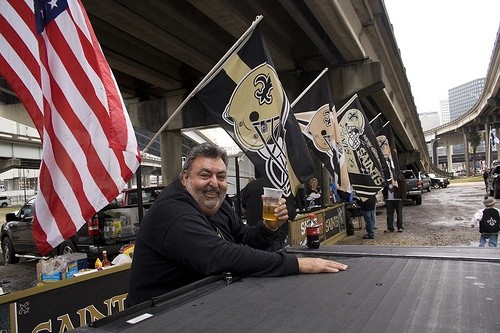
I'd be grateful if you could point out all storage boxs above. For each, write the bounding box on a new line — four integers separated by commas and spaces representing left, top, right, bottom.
36, 256, 78, 282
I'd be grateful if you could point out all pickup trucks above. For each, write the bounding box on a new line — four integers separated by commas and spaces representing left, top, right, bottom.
399, 170, 423, 205
0, 194, 146, 266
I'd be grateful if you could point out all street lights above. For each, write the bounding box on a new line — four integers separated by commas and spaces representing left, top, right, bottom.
431, 138, 441, 164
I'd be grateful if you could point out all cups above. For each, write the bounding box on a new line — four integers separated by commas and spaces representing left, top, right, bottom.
262, 187, 283, 221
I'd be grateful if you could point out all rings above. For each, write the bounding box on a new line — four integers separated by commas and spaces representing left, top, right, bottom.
285, 209, 288, 214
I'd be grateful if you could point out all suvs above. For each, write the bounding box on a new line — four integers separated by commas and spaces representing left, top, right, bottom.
120, 185, 166, 211
485, 165, 500, 199
0, 196, 12, 208
412, 170, 432, 194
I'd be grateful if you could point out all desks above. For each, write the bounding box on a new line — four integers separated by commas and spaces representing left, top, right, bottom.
68, 245, 500, 333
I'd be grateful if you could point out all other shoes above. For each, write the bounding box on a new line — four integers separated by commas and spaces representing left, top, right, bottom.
347, 231, 354, 235
362, 234, 375, 239
397, 229, 404, 232
384, 229, 394, 233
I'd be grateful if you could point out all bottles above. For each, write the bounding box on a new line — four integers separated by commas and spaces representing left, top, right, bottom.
101, 251, 111, 267
305, 213, 320, 249
95, 258, 103, 269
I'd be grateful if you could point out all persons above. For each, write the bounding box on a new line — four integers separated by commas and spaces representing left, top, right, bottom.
471, 196, 500, 247
305, 178, 322, 205
241, 178, 297, 251
125, 142, 349, 309
483, 167, 489, 192
382, 174, 407, 232
329, 176, 378, 239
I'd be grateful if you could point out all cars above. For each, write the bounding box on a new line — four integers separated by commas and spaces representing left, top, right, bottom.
426, 173, 451, 189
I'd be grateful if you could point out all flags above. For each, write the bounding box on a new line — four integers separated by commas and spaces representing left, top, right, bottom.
337, 98, 401, 202
293, 69, 351, 194
195, 24, 316, 221
0, 0, 141, 254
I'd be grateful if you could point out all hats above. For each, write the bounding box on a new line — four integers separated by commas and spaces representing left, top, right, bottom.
483, 196, 496, 206
